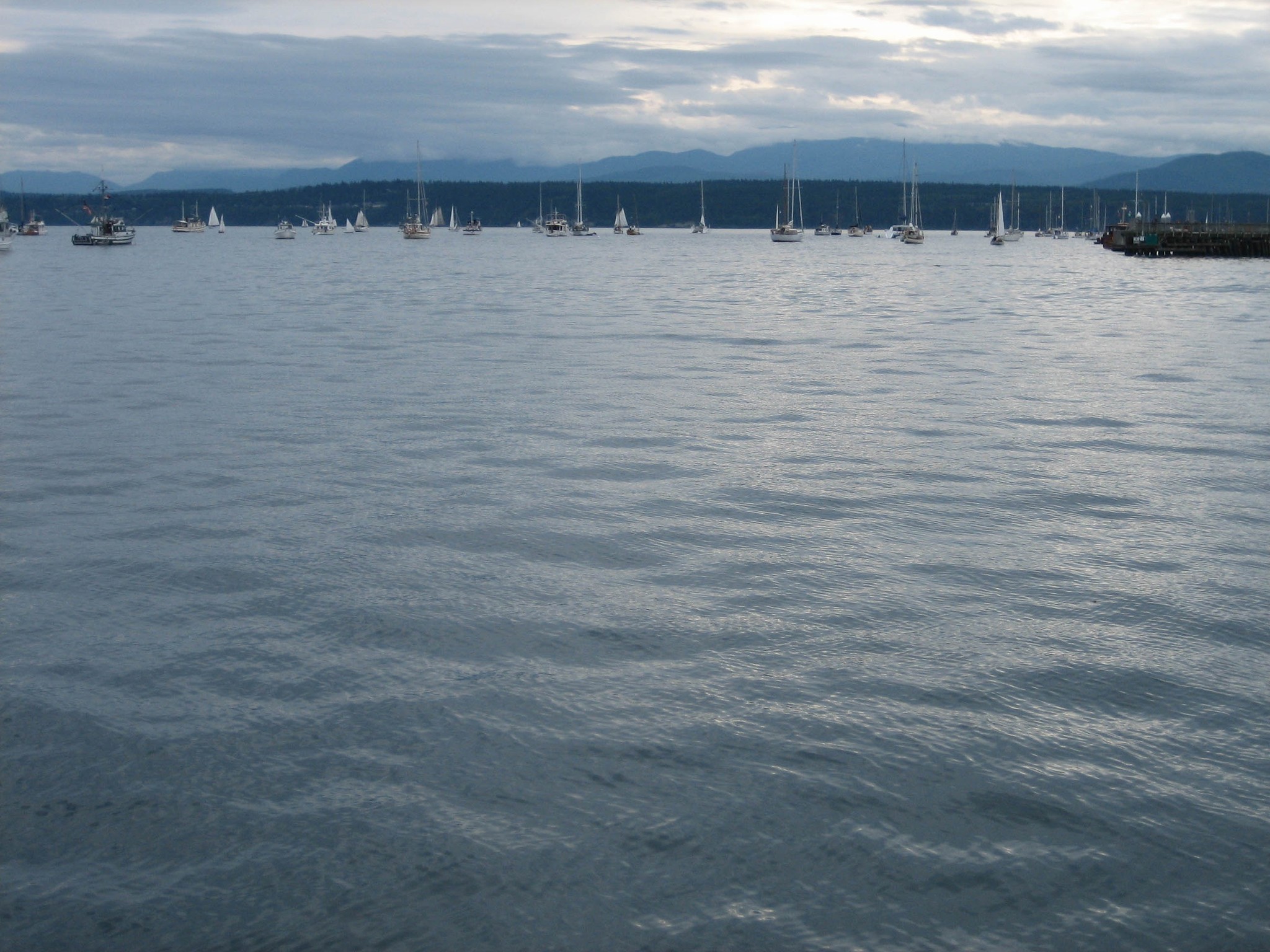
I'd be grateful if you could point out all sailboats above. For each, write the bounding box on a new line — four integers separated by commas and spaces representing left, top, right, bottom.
691, 180, 710, 234
429, 207, 449, 228
569, 167, 594, 236
770, 138, 805, 243
985, 166, 1110, 246
344, 218, 355, 233
397, 139, 431, 240
448, 204, 461, 231
353, 189, 371, 232
612, 193, 628, 234
217, 214, 225, 233
17, 176, 49, 236
814, 137, 960, 243
525, 183, 545, 233
208, 206, 220, 228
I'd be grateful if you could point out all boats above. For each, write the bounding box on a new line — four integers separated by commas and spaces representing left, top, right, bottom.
302, 219, 308, 228
462, 211, 482, 235
53, 179, 138, 246
273, 214, 297, 239
295, 200, 337, 235
626, 208, 641, 235
171, 199, 207, 232
517, 221, 524, 228
543, 199, 569, 238
0, 205, 19, 250
